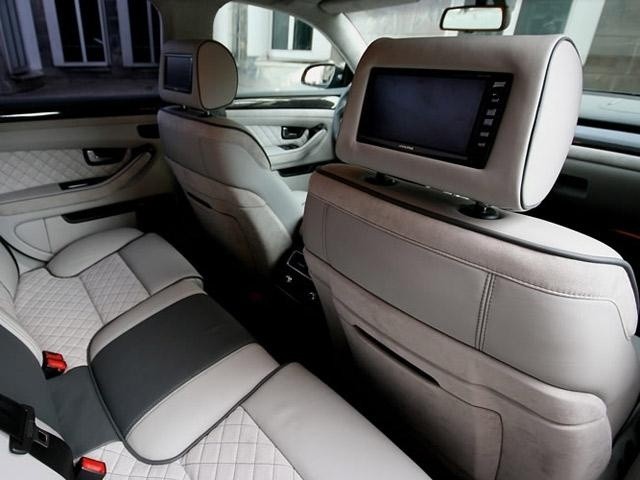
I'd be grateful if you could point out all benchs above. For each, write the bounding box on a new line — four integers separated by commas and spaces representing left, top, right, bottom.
0, 228, 438, 479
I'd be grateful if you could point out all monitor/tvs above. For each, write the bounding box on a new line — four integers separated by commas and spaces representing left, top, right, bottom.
355, 66, 514, 169
164, 54, 193, 94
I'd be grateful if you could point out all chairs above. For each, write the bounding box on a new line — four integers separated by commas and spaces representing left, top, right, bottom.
294, 35, 639, 480
156, 36, 319, 282
